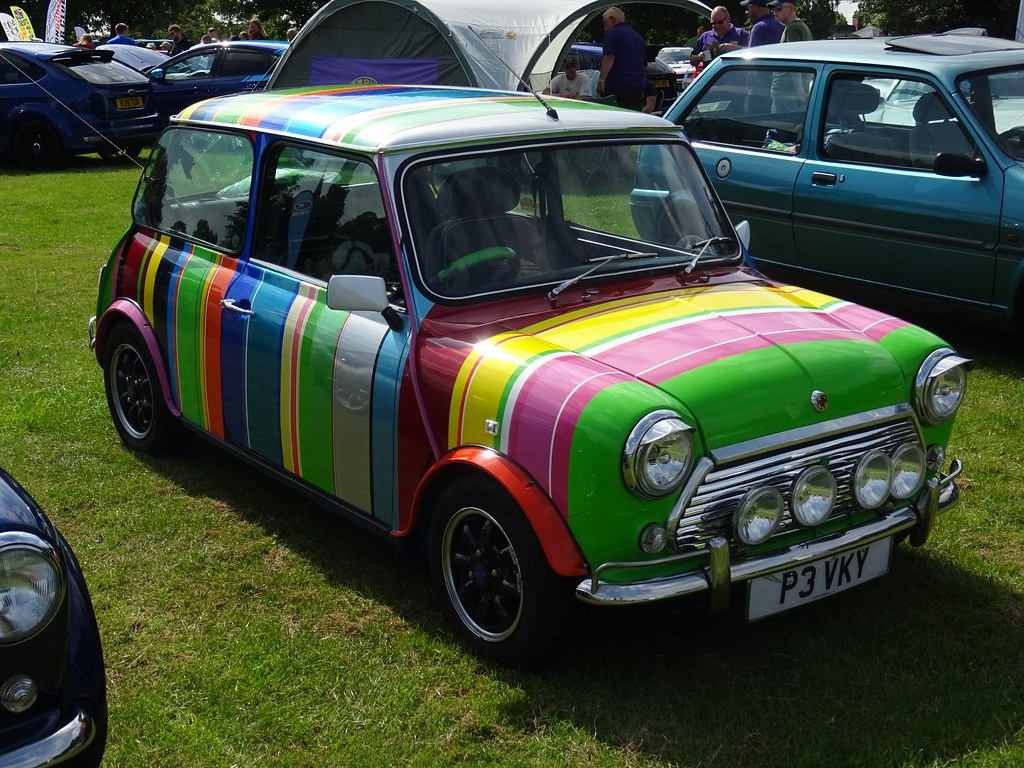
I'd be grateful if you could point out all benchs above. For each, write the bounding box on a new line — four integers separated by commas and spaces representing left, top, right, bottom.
169, 179, 383, 248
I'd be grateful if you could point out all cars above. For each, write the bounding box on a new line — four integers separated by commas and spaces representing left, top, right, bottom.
631, 25, 1024, 336
88, 84, 975, 668
0, 42, 161, 172
95, 41, 290, 133
559, 43, 678, 116
656, 47, 696, 90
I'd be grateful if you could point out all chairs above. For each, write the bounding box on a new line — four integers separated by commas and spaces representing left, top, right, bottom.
826, 82, 903, 168
909, 89, 975, 163
581, 88, 665, 116
421, 164, 538, 297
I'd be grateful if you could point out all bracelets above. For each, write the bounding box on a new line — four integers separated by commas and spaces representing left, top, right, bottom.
597, 79, 604, 83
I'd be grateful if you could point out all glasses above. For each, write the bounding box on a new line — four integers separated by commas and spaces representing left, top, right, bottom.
746, 6, 755, 11
169, 31, 176, 35
709, 15, 728, 26
772, 4, 794, 11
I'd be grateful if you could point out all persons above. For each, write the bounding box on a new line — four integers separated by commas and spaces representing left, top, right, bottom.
718, 0, 786, 114
767, 0, 813, 114
72, 18, 299, 57
689, 6, 750, 113
596, 7, 658, 114
542, 56, 592, 100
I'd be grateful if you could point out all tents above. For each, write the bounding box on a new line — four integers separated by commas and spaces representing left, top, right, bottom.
252, 0, 713, 96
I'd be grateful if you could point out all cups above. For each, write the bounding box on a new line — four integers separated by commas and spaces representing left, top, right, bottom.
701, 50, 713, 61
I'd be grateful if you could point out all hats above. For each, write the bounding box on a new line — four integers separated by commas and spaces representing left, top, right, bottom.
146, 42, 156, 48
563, 56, 577, 69
766, 0, 796, 10
740, 0, 770, 7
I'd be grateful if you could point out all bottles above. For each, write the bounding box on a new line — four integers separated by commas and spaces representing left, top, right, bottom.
713, 41, 722, 52
